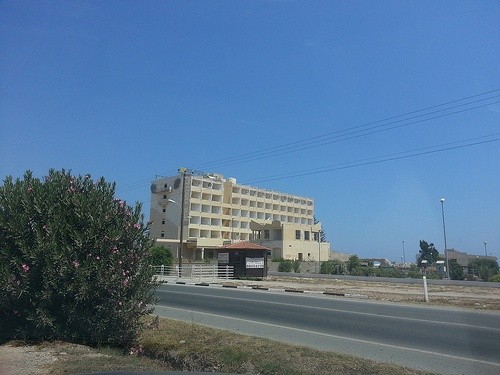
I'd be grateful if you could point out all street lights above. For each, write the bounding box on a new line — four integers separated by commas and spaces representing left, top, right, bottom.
440, 198, 450, 280
167, 198, 184, 278
484, 240, 487, 257
